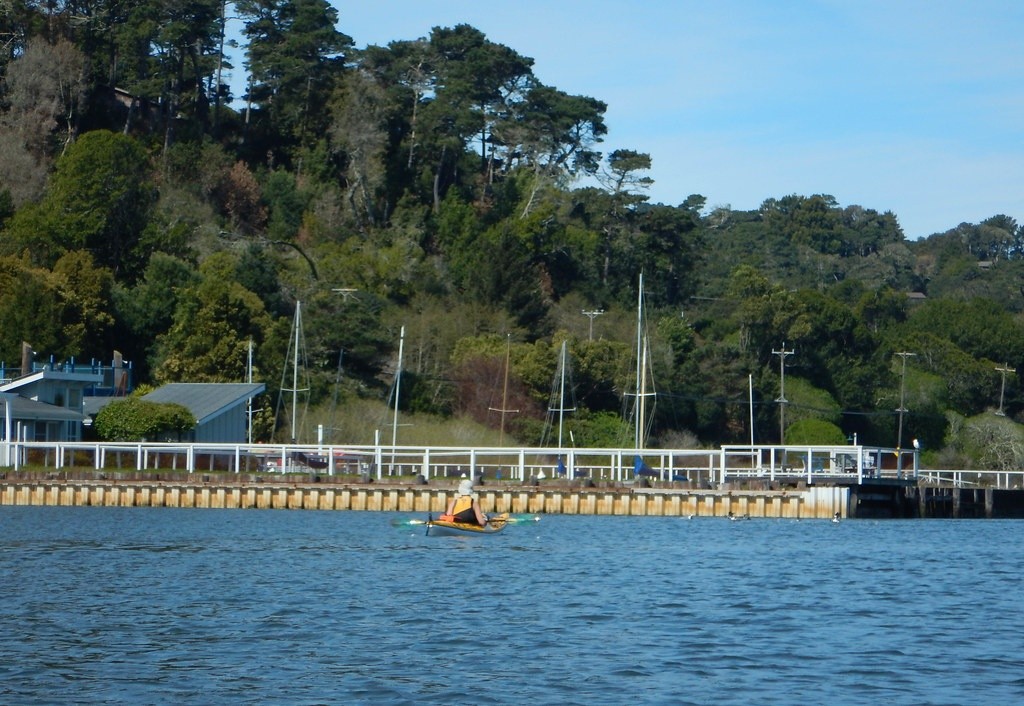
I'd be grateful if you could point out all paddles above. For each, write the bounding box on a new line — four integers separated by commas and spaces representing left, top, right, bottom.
390, 513, 542, 531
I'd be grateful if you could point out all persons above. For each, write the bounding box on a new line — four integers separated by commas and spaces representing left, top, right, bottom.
446, 479, 486, 525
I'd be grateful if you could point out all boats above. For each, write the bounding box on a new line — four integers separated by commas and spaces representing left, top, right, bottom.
427, 512, 513, 538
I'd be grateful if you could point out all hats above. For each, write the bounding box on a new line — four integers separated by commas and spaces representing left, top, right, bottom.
458, 480, 474, 496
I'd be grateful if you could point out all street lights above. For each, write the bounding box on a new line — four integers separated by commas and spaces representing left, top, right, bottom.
581, 309, 604, 340
772, 341, 795, 444
893, 351, 916, 479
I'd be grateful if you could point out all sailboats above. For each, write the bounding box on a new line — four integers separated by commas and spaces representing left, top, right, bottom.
254, 301, 334, 475
